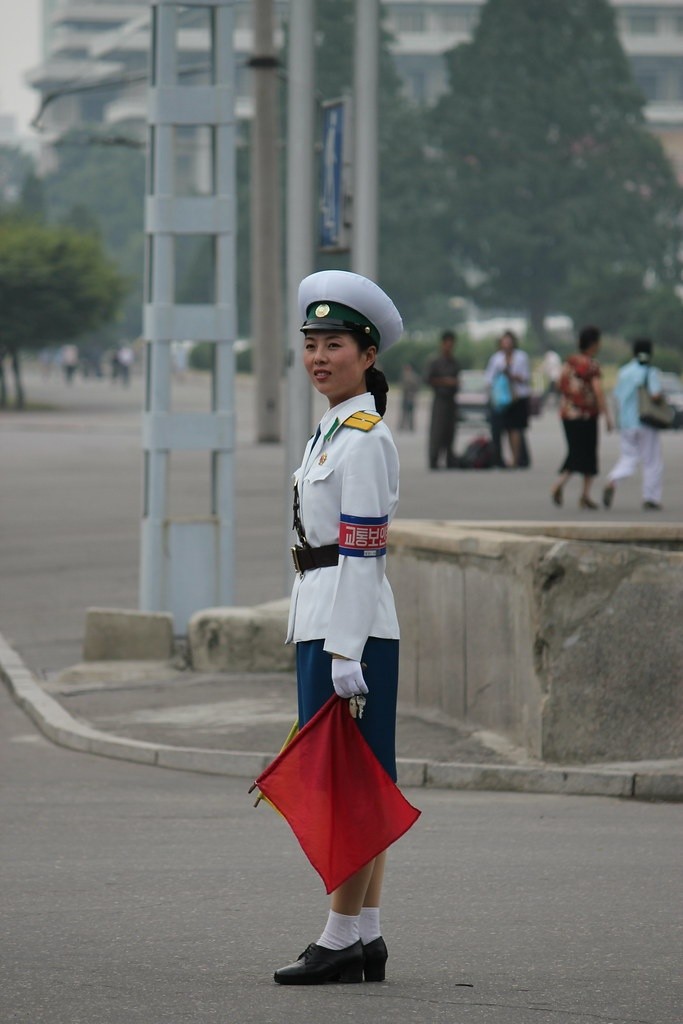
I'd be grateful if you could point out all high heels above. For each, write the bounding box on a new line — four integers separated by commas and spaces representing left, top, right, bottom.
274, 937, 388, 984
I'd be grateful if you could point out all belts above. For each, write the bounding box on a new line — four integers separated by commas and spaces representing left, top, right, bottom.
290, 542, 340, 574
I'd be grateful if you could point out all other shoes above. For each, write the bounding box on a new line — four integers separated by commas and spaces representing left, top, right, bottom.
430, 459, 437, 469
580, 495, 598, 509
642, 502, 663, 510
553, 487, 562, 505
448, 457, 460, 467
602, 486, 613, 506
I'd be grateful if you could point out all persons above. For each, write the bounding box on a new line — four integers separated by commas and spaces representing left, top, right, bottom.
248, 266, 412, 990
598, 330, 668, 516
532, 344, 568, 418
423, 329, 462, 466
33, 329, 202, 405
480, 325, 536, 471
549, 325, 604, 513
391, 360, 423, 434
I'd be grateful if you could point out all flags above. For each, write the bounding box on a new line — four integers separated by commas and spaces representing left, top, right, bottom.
256, 689, 423, 896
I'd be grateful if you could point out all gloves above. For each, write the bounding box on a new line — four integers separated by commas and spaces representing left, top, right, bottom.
331, 657, 370, 699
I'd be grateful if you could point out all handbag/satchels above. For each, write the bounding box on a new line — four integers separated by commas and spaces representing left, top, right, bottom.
638, 366, 677, 427
490, 369, 514, 416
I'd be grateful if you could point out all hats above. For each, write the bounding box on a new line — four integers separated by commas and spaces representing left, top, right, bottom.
298, 270, 405, 356
